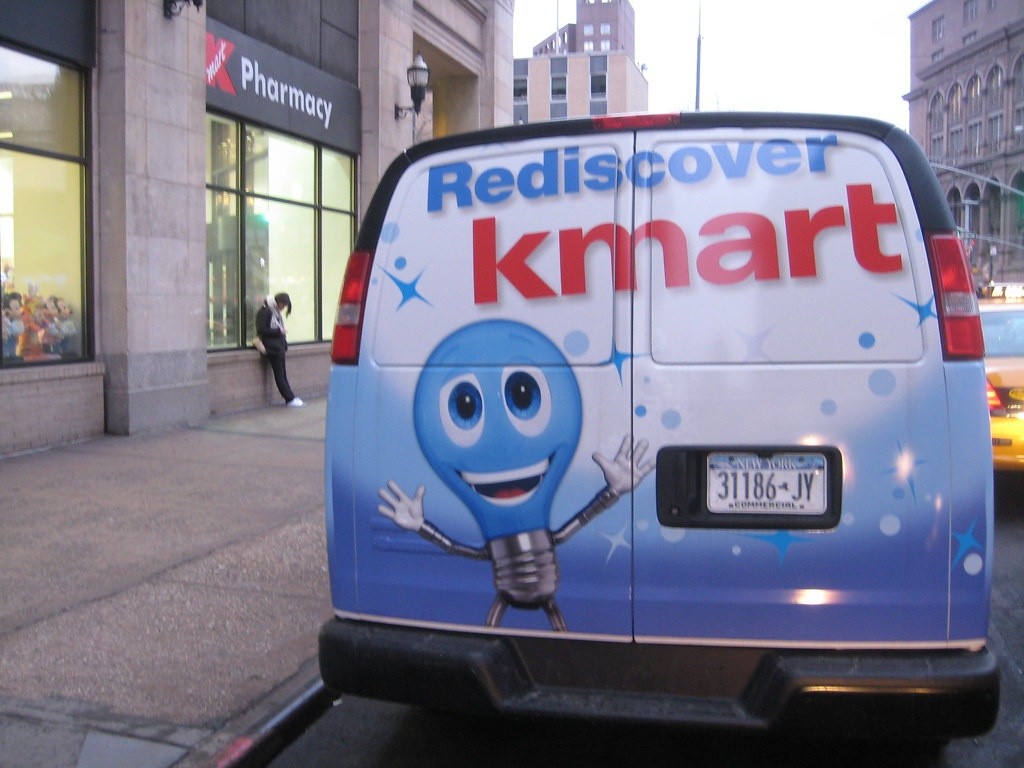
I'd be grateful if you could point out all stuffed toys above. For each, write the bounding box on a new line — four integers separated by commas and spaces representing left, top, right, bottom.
0, 291, 78, 367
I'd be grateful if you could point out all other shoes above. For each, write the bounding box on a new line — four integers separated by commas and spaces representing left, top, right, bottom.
287, 397, 305, 407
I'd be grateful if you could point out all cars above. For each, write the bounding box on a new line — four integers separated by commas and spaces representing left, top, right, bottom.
975, 281, 1024, 475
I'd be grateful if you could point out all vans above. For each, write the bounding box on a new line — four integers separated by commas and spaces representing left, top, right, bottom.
321, 111, 1007, 768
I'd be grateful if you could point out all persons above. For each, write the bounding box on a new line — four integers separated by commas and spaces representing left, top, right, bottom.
255, 293, 306, 407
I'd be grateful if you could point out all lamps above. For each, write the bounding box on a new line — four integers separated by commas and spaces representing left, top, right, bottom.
394, 49, 430, 122
163, 0, 204, 20
